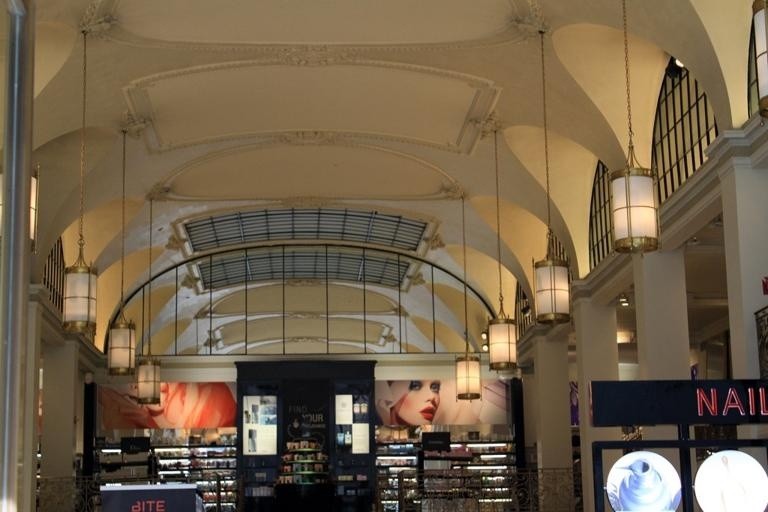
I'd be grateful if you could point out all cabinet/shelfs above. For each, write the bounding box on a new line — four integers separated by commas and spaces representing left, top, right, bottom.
87, 444, 239, 511
373, 440, 521, 511
273, 448, 335, 511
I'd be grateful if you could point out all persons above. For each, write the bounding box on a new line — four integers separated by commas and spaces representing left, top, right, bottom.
375, 380, 441, 426
119, 382, 237, 427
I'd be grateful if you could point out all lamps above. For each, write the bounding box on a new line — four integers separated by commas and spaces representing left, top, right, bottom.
134, 194, 163, 406
531, 27, 571, 327
106, 129, 137, 377
62, 25, 100, 340
455, 190, 483, 403
487, 128, 519, 375
610, 2, 660, 258
